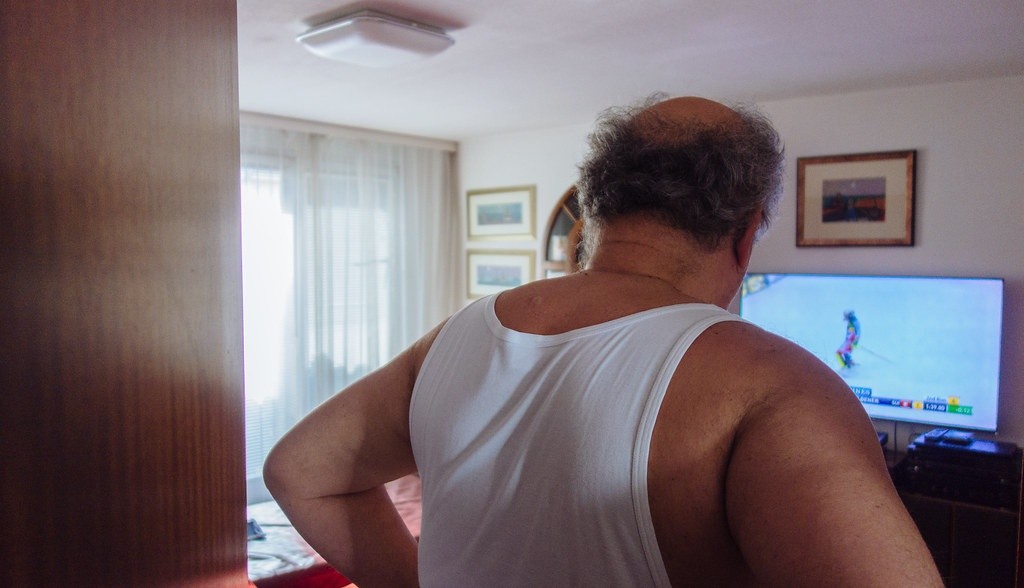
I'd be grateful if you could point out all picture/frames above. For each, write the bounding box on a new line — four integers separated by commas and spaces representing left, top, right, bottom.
467, 249, 537, 299
797, 150, 916, 248
465, 184, 536, 244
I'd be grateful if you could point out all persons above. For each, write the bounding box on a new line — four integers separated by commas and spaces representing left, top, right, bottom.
262, 94, 947, 588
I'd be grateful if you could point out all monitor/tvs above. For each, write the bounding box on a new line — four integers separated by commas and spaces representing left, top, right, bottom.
740, 273, 1005, 432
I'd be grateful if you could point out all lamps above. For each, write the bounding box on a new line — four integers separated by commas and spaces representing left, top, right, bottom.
297, 10, 454, 70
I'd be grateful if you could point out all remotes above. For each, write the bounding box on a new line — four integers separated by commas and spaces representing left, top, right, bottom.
925, 429, 949, 441
